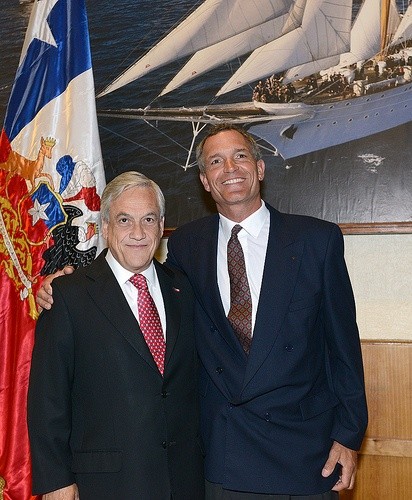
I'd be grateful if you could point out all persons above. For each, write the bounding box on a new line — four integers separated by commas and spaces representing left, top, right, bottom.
35, 122, 369, 500
25, 171, 207, 500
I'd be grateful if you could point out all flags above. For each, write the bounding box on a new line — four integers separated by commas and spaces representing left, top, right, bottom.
0, 0, 110, 498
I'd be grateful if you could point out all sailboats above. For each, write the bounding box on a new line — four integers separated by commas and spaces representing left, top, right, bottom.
95, 0, 412, 160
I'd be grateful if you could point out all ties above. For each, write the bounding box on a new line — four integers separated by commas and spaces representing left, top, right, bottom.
128, 274, 166, 379
225, 225, 253, 358
